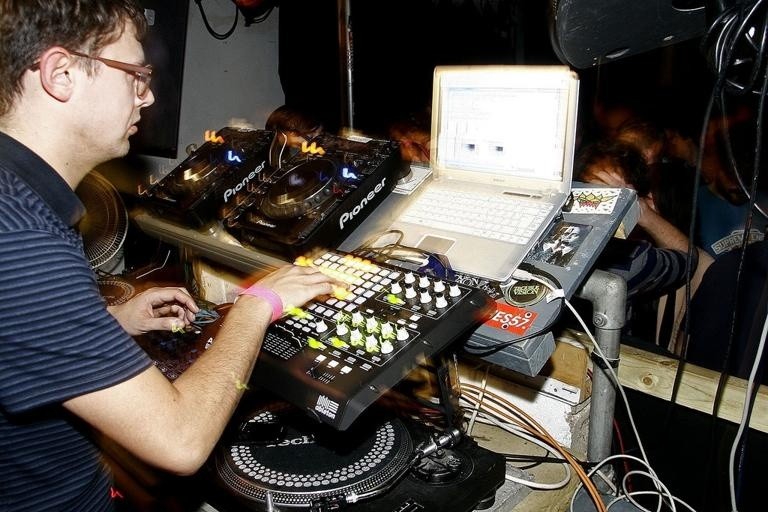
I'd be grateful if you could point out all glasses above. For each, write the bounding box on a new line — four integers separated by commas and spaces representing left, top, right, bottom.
30, 50, 156, 102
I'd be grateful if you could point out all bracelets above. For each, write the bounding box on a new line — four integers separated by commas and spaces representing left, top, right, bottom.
232, 283, 287, 322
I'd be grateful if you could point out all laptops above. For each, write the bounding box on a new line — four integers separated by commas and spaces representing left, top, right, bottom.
369, 61, 581, 286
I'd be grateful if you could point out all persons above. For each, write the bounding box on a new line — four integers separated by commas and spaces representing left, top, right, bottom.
1, 0, 337, 511
265, 89, 768, 388
